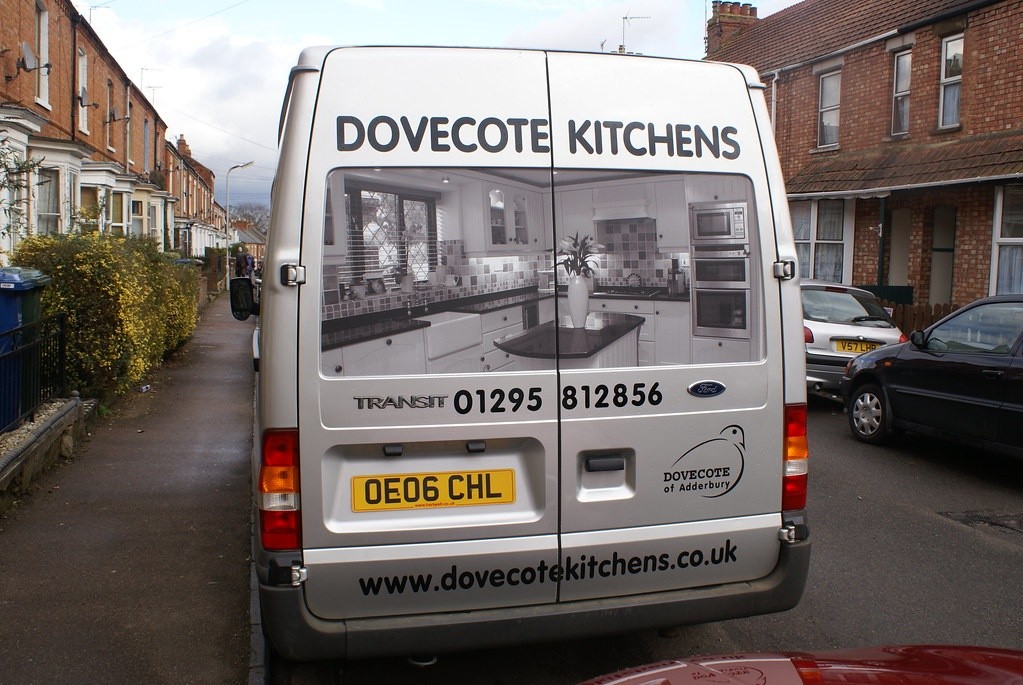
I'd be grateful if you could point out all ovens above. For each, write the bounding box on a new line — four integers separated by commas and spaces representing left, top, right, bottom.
691, 244, 751, 339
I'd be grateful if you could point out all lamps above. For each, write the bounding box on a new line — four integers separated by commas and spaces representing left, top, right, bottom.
442, 176, 450, 183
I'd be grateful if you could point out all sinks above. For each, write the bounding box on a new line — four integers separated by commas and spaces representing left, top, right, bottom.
411, 311, 483, 360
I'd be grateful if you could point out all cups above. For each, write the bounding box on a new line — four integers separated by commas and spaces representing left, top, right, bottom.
400, 276, 418, 293
492, 218, 502, 224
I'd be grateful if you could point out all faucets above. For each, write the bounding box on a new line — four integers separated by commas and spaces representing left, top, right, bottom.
411, 286, 420, 307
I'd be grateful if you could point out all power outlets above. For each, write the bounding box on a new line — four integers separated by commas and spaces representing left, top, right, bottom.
649, 278, 659, 284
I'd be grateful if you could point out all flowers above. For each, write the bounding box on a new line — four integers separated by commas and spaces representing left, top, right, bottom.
549, 230, 605, 278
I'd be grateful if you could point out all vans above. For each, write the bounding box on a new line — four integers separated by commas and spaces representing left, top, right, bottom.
229, 45, 812, 668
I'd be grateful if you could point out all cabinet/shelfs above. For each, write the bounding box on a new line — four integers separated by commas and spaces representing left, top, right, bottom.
692, 336, 752, 364
530, 185, 547, 251
652, 174, 690, 253
654, 301, 691, 366
341, 327, 426, 375
560, 183, 597, 246
461, 181, 531, 257
589, 299, 654, 367
544, 186, 565, 250
482, 306, 525, 373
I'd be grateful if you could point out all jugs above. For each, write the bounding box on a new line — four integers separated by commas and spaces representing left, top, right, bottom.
428, 265, 459, 287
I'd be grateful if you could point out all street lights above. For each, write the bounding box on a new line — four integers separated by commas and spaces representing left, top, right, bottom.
225, 161, 254, 290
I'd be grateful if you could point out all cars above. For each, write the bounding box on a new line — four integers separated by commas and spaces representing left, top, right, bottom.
800, 278, 909, 404
838, 294, 1023, 461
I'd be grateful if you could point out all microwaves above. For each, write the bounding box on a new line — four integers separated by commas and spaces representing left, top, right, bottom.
692, 207, 746, 239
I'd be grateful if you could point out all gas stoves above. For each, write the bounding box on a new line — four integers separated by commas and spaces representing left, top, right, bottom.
593, 286, 661, 297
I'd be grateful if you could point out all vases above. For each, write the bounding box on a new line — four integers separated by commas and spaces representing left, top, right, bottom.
566, 274, 590, 329
